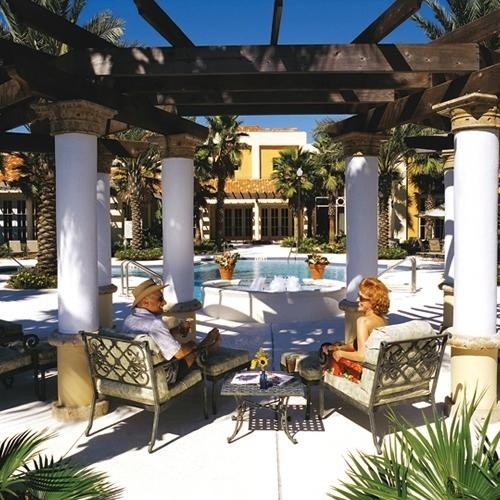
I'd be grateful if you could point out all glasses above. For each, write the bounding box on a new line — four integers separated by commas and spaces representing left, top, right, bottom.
358, 293, 371, 302
159, 297, 165, 303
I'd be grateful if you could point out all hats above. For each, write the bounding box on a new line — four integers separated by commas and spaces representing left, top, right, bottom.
130, 278, 169, 309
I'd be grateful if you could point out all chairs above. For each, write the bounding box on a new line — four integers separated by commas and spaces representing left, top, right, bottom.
1, 316, 209, 453
317, 319, 454, 454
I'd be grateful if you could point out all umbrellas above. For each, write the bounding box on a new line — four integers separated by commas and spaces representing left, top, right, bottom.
415, 203, 445, 239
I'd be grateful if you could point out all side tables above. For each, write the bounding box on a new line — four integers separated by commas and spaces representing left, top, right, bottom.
219, 370, 305, 444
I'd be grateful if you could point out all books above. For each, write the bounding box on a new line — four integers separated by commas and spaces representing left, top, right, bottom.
230, 373, 261, 385
265, 373, 296, 388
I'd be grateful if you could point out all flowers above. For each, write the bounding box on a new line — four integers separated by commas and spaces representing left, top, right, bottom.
249, 346, 274, 372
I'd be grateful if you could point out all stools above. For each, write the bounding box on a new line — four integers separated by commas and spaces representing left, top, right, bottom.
198, 345, 251, 416
280, 351, 324, 421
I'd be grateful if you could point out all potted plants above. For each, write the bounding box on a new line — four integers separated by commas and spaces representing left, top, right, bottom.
304, 252, 330, 281
215, 251, 240, 280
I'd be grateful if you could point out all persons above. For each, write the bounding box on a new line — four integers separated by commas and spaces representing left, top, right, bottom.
319, 277, 390, 381
122, 279, 221, 386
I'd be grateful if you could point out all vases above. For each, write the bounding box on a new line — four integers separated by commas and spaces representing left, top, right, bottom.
259, 372, 270, 389
